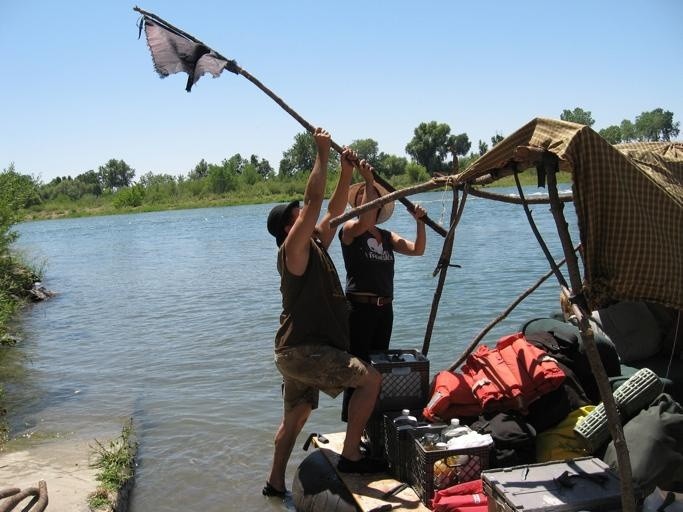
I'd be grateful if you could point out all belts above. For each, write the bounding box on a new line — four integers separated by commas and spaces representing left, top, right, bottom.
345, 292, 393, 307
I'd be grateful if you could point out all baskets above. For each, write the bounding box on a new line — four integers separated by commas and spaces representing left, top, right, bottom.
365, 345, 432, 411
381, 412, 449, 483
409, 432, 502, 509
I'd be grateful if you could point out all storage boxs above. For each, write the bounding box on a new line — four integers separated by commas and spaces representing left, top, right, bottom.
480, 455, 644, 512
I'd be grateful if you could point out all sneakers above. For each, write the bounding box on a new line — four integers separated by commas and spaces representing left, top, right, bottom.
336, 453, 385, 475
261, 480, 294, 503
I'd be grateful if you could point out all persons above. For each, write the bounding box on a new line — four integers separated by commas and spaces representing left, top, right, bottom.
264, 127, 382, 496
339, 158, 427, 423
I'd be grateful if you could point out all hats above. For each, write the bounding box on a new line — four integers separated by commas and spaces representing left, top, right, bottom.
266, 198, 300, 248
348, 179, 396, 224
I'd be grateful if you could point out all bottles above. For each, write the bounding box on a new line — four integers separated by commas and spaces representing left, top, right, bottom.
394, 408, 481, 490
